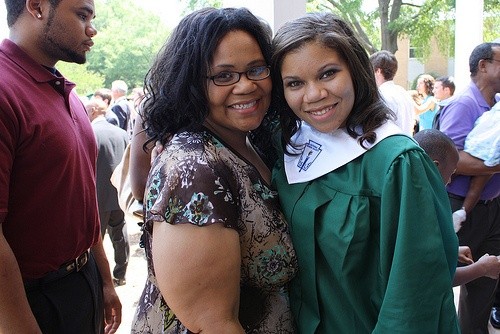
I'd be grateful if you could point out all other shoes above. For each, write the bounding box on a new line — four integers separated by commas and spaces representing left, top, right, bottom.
489, 306, 500, 328
112, 278, 125, 286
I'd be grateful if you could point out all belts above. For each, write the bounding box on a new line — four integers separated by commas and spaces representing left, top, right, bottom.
31, 249, 89, 288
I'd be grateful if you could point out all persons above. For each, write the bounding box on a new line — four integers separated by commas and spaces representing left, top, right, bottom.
129, 6, 300, 334
407, 73, 440, 131
367, 50, 417, 139
409, 127, 500, 289
437, 42, 500, 334
110, 79, 131, 133
78, 96, 131, 288
91, 88, 121, 127
447, 89, 499, 234
264, 12, 461, 334
432, 75, 457, 132
0, 0, 122, 334
128, 86, 152, 134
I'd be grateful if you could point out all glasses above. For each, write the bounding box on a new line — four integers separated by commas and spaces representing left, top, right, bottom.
206, 66, 271, 87
